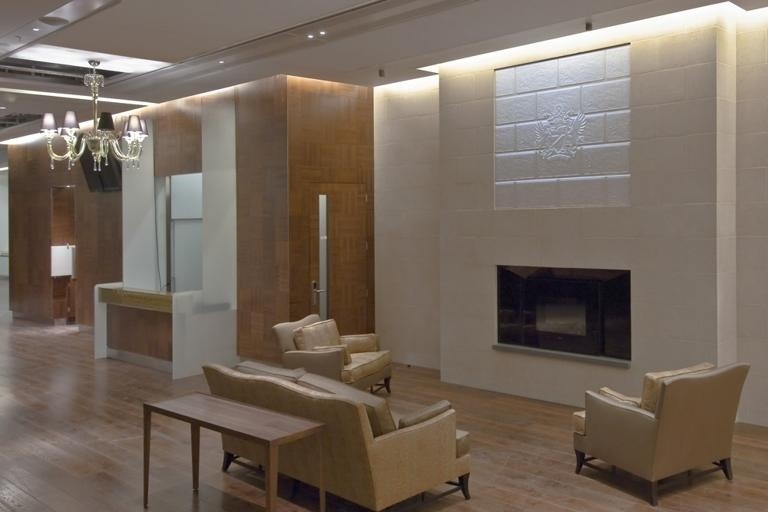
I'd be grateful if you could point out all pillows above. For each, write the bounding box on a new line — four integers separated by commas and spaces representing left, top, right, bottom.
296, 319, 343, 358
297, 374, 394, 436
642, 362, 715, 415
234, 361, 301, 382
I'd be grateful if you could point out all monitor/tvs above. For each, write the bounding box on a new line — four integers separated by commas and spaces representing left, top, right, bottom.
492, 265, 632, 368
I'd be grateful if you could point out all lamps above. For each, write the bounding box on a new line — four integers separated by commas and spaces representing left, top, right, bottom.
40, 58, 148, 172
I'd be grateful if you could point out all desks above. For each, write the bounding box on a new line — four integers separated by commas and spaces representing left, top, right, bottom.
141, 391, 327, 512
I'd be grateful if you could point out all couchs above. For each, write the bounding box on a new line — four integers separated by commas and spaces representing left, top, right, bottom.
571, 362, 750, 506
201, 362, 471, 512
271, 314, 392, 396
